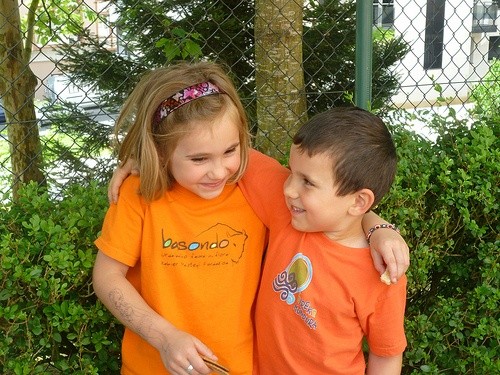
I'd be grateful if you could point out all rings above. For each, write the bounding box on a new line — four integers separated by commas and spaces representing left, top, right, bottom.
186, 365, 194, 374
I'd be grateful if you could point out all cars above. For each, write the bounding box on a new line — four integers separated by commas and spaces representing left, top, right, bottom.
35, 104, 84, 127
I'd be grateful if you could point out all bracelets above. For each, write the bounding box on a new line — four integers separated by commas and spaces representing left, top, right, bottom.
366, 224, 400, 245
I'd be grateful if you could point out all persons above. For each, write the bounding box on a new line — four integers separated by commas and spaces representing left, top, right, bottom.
108, 107, 408, 375
92, 62, 410, 375
